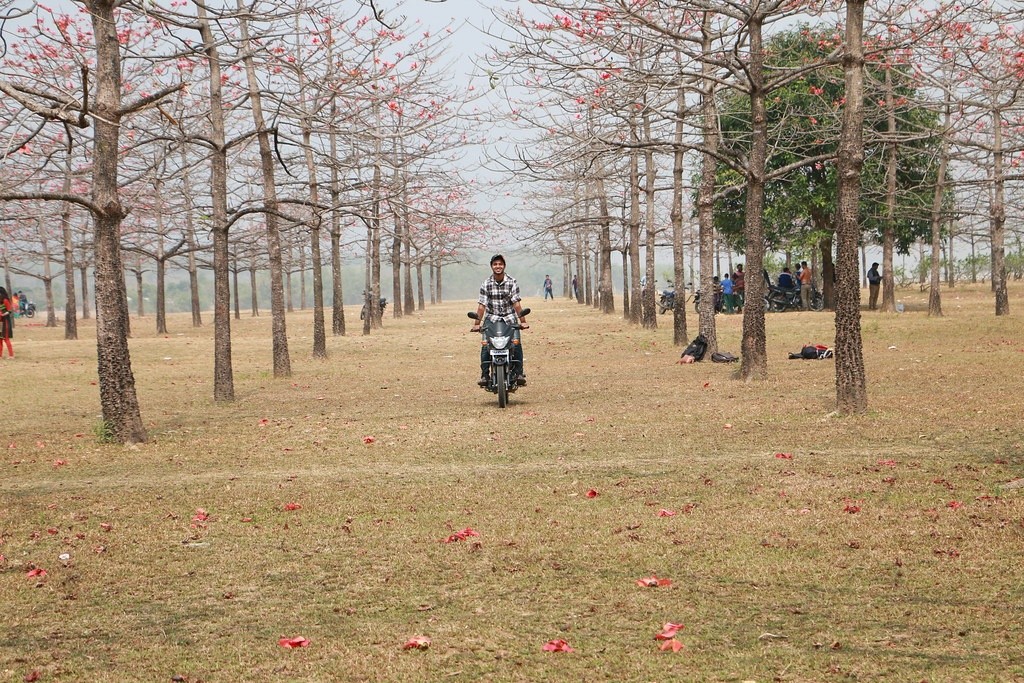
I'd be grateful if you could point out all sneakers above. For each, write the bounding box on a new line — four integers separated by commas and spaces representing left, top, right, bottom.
873, 307, 878, 310
0, 356, 3, 359
516, 374, 526, 385
869, 307, 874, 310
481, 373, 490, 384
9, 355, 14, 359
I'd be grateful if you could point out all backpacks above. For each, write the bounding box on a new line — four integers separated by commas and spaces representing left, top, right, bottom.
711, 351, 739, 363
788, 344, 833, 360
679, 335, 708, 365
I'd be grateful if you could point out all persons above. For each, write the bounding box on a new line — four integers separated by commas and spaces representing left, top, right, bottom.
472, 254, 529, 385
713, 264, 745, 315
867, 262, 883, 310
544, 275, 553, 302
572, 275, 578, 298
641, 276, 647, 297
0, 286, 28, 359
763, 261, 814, 311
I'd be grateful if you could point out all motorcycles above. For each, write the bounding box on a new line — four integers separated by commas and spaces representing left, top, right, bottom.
656, 279, 692, 314
467, 308, 531, 407
693, 269, 825, 314
20, 303, 36, 318
360, 290, 388, 320
